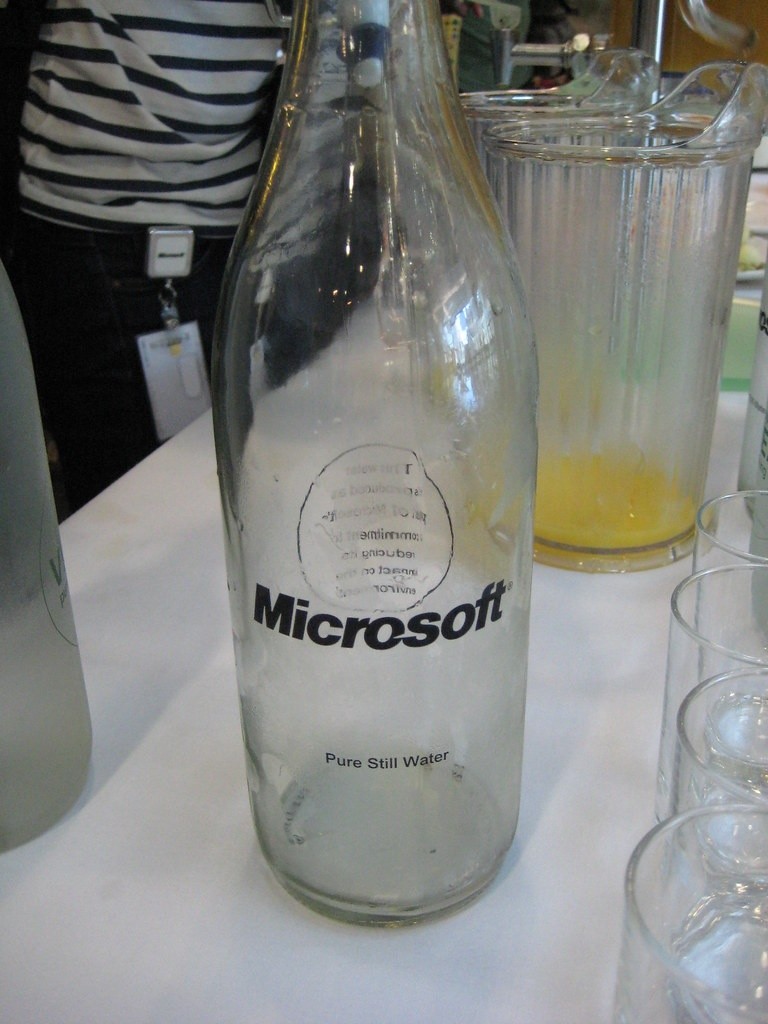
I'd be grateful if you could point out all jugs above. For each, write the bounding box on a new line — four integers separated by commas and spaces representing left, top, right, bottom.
480, 63, 768, 571
457, 48, 659, 185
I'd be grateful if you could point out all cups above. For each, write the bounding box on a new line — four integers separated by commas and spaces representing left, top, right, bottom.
611, 488, 768, 1024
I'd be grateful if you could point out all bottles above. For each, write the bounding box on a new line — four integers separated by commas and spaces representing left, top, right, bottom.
213, 0, 540, 924
1, 259, 93, 853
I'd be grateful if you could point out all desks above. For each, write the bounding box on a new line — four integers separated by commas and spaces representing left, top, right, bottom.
0, 275, 768, 1024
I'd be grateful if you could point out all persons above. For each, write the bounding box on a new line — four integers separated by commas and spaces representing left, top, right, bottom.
0, 1, 485, 524
